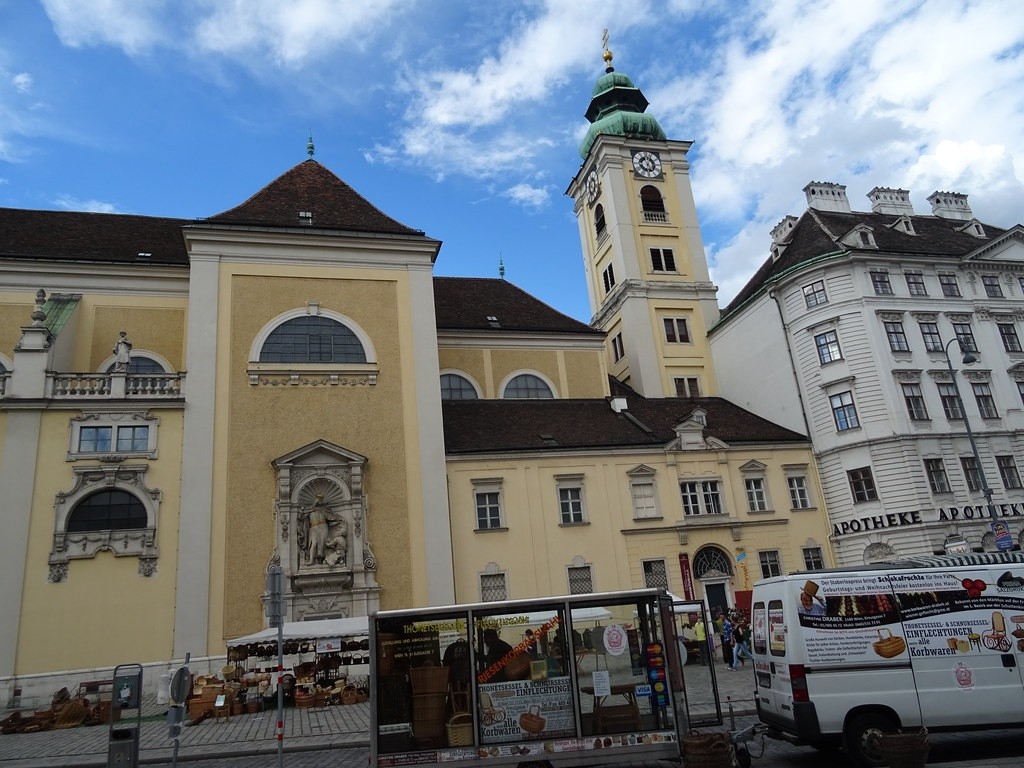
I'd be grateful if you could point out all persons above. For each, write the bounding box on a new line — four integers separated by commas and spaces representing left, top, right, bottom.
475, 628, 526, 683
694, 616, 710, 666
716, 613, 752, 672
299, 494, 344, 566
798, 592, 827, 615
553, 629, 563, 655
112, 328, 134, 373
524, 628, 538, 658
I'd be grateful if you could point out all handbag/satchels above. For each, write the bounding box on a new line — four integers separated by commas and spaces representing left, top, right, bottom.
449, 639, 470, 659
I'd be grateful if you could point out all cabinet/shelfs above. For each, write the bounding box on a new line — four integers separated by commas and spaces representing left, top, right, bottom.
79, 680, 121, 722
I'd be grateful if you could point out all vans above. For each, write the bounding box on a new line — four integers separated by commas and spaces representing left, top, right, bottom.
750, 562, 1023, 768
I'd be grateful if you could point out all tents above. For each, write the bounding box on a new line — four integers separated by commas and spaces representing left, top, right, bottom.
480, 605, 614, 628
632, 589, 709, 619
224, 607, 611, 687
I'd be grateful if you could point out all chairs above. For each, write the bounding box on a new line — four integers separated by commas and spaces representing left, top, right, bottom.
215, 689, 231, 723
571, 626, 610, 674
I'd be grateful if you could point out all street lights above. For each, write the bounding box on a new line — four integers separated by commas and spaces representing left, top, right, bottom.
945, 337, 1012, 553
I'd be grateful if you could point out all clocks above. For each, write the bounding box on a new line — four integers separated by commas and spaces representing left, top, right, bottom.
587, 171, 598, 202
633, 152, 661, 178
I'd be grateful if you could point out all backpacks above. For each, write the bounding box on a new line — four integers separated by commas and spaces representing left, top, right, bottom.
739, 623, 751, 642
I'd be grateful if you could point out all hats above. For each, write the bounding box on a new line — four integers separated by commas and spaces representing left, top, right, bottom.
800, 580, 820, 600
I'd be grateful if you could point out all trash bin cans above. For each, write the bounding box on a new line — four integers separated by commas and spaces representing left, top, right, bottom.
106, 663, 145, 768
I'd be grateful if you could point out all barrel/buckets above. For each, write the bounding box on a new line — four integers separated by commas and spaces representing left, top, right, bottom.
222, 665, 236, 678
947, 638, 957, 650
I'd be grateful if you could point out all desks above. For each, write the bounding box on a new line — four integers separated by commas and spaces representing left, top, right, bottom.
581, 682, 648, 735
575, 649, 598, 672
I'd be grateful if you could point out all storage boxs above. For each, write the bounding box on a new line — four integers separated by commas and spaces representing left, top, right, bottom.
410, 667, 449, 694
202, 684, 223, 699
189, 698, 216, 720
413, 694, 443, 736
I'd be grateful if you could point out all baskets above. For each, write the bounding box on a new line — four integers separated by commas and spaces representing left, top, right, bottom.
872, 628, 905, 658
221, 661, 236, 678
245, 693, 261, 713
232, 699, 245, 714
519, 705, 545, 734
409, 665, 450, 742
448, 721, 475, 747
293, 661, 366, 709
603, 733, 676, 747
250, 638, 369, 663
681, 730, 735, 768
502, 649, 532, 680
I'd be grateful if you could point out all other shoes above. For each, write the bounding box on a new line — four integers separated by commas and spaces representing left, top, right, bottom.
725, 666, 737, 671
